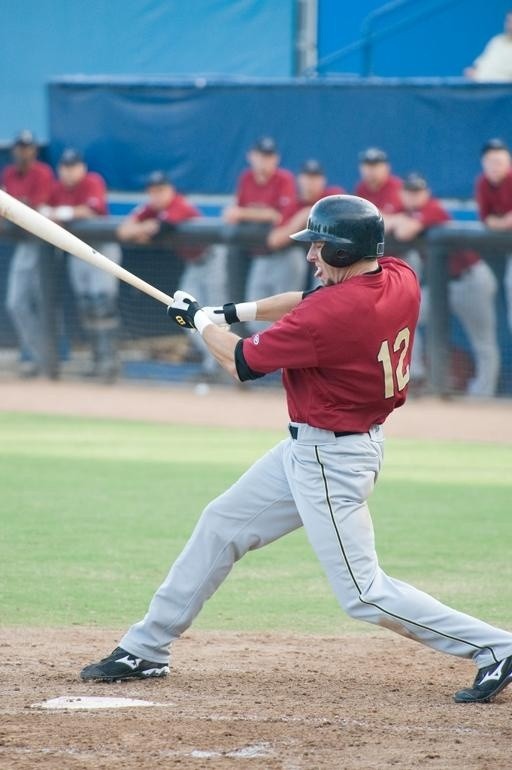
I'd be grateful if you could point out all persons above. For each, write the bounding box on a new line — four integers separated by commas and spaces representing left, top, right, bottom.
80, 195, 512, 702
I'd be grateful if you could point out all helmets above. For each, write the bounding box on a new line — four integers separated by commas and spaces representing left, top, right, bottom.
289, 195, 384, 266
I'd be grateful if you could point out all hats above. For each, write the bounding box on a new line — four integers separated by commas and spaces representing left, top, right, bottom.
253, 137, 277, 151
405, 172, 427, 191
359, 148, 386, 163
15, 132, 36, 147
147, 171, 167, 185
481, 138, 508, 153
60, 149, 79, 164
301, 160, 323, 174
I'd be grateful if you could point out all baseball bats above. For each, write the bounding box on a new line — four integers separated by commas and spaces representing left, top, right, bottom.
0, 189, 175, 306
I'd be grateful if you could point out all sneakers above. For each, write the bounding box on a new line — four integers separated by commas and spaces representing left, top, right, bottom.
455, 655, 512, 702
80, 647, 170, 682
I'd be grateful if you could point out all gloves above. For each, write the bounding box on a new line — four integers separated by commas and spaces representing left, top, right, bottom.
167, 291, 201, 330
201, 303, 240, 327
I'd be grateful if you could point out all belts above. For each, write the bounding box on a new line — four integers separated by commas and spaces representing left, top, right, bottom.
290, 426, 357, 439
